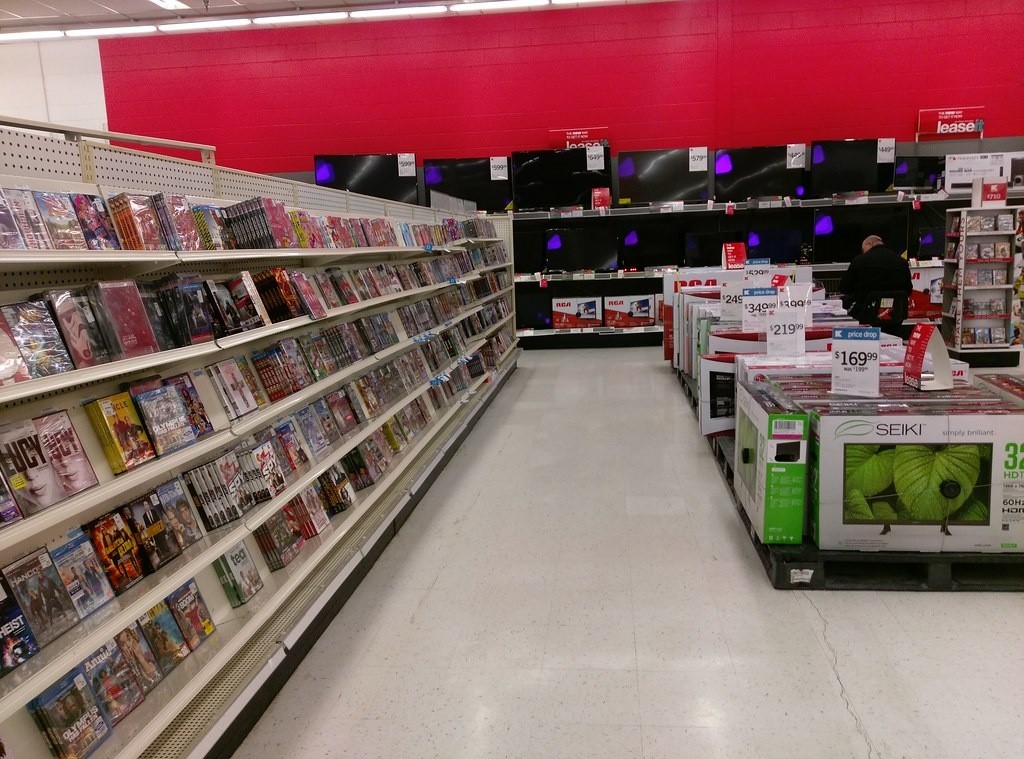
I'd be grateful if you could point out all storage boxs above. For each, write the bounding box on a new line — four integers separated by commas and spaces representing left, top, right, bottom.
663, 269, 1024, 554
944, 151, 1024, 194
973, 177, 1007, 208
551, 298, 603, 328
604, 295, 655, 327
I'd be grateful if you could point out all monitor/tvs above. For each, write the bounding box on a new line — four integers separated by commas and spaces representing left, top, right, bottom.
313, 139, 973, 331
629, 299, 650, 318
843, 442, 992, 535
577, 300, 596, 319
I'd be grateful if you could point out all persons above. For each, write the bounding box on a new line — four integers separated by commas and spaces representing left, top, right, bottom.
27, 587, 49, 634
174, 594, 209, 643
98, 523, 129, 581
139, 500, 173, 563
164, 503, 193, 551
70, 567, 95, 615
838, 235, 913, 337
116, 628, 157, 685
36, 570, 68, 626
80, 560, 104, 596
174, 499, 203, 542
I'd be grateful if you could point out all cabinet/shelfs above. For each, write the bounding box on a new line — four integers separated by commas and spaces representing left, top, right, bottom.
1, 120, 513, 759
943, 205, 1024, 355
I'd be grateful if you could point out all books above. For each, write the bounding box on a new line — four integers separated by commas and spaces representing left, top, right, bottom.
0, 188, 497, 248
0, 244, 514, 759
